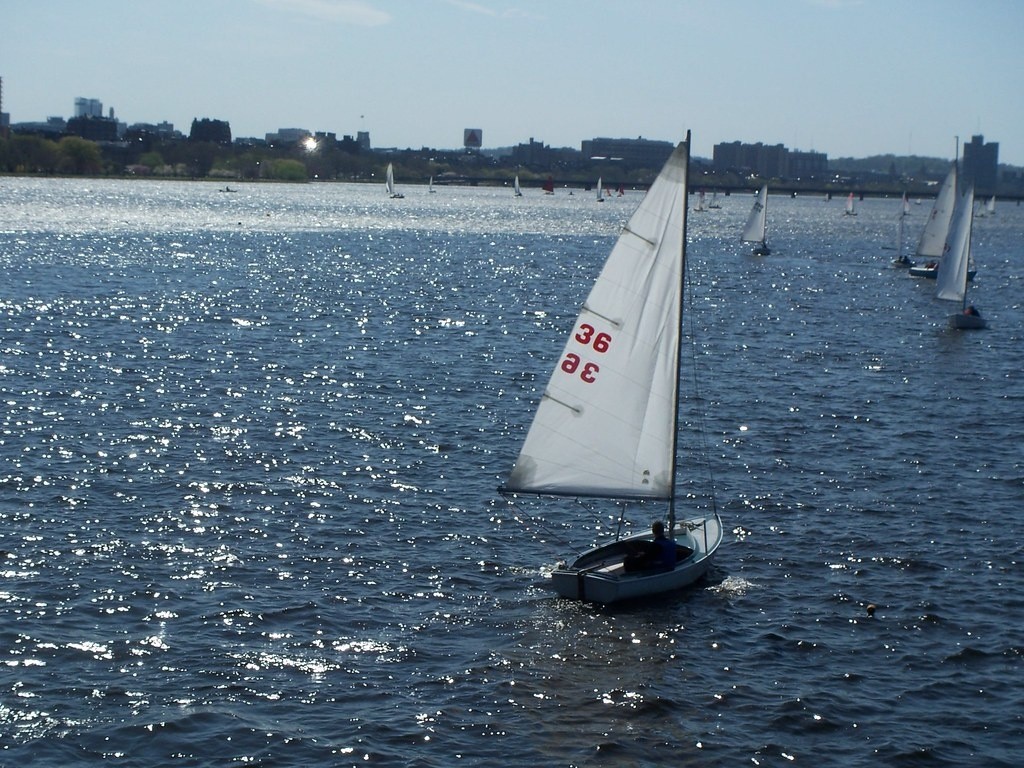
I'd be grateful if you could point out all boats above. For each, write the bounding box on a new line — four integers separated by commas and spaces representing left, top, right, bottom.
220, 189, 238, 193
892, 260, 918, 268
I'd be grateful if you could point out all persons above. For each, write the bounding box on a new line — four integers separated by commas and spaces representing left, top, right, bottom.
630, 521, 676, 574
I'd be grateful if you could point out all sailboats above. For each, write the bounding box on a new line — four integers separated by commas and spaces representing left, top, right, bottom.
842, 191, 859, 217
514, 175, 522, 197
910, 160, 978, 281
741, 183, 771, 255
497, 128, 726, 605
931, 181, 988, 329
974, 194, 997, 217
693, 185, 722, 213
615, 183, 625, 197
427, 176, 436, 193
596, 176, 605, 202
541, 175, 554, 194
384, 161, 404, 198
899, 190, 911, 216
